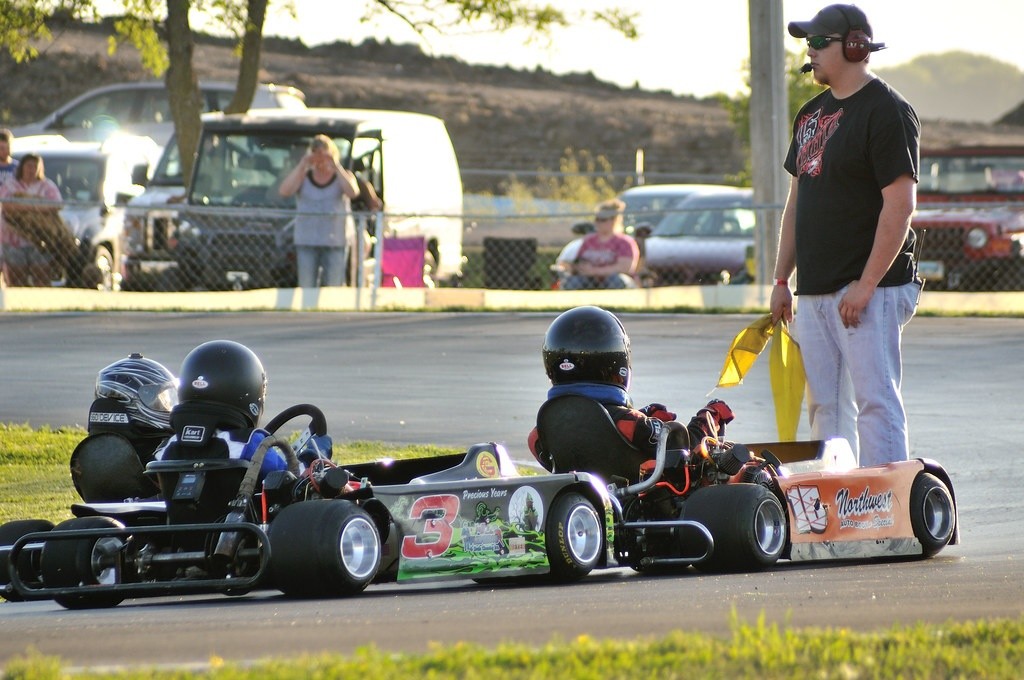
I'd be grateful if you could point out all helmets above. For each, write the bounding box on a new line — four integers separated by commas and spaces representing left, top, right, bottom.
177, 340, 267, 428
543, 306, 632, 390
95, 353, 182, 429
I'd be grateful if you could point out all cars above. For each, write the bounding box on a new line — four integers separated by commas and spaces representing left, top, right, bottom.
554, 183, 757, 290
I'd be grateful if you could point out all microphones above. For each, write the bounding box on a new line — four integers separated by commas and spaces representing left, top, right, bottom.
800, 63, 813, 73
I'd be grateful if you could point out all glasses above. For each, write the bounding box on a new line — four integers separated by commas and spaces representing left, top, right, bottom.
595, 216, 616, 222
805, 35, 845, 50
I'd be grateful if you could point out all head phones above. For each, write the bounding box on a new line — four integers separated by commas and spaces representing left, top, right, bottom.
834, 4, 885, 63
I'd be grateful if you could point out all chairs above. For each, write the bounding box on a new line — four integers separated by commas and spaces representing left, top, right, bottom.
486, 237, 546, 290
143, 460, 252, 504
234, 152, 273, 206
380, 239, 442, 289
536, 394, 682, 482
69, 432, 161, 502
551, 219, 652, 290
707, 218, 742, 235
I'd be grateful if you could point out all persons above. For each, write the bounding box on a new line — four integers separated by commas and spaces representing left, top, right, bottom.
771, 2, 928, 468
280, 134, 383, 288
556, 224, 596, 290
154, 335, 336, 520
528, 306, 763, 521
0, 128, 64, 289
91, 356, 184, 499
233, 154, 290, 207
565, 198, 640, 289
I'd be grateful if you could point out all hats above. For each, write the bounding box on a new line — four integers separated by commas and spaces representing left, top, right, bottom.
788, 4, 874, 41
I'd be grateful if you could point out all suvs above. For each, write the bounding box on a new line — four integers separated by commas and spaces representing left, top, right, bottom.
911, 144, 1024, 291
0, 81, 464, 291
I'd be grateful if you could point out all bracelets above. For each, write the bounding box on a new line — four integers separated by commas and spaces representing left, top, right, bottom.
773, 278, 788, 285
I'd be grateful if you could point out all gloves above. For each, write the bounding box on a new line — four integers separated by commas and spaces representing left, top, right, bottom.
695, 399, 735, 428
637, 402, 678, 423
297, 433, 333, 466
